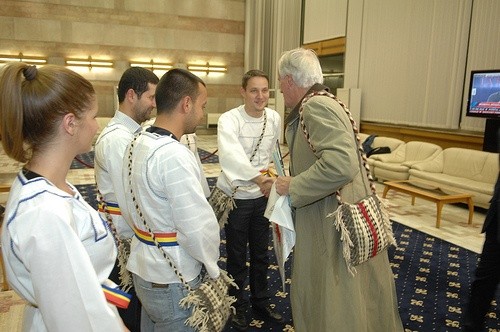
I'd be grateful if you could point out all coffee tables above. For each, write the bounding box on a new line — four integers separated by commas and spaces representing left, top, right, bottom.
383, 180, 476, 228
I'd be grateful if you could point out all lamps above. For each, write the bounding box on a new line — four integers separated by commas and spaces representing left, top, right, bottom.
129, 59, 175, 71
65, 55, 115, 69
187, 63, 229, 74
0, 52, 48, 64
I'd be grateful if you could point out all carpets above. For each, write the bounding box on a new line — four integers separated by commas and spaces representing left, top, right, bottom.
70, 183, 500, 332
69, 147, 219, 169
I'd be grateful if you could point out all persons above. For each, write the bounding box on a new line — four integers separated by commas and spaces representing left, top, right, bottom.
259, 48, 403, 332
0, 62, 130, 332
461, 167, 500, 332
122, 69, 221, 332
95, 66, 160, 332
214, 69, 283, 332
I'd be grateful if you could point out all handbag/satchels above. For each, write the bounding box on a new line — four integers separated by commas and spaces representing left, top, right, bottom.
327, 193, 397, 275
206, 186, 237, 228
178, 268, 239, 332
117, 237, 133, 292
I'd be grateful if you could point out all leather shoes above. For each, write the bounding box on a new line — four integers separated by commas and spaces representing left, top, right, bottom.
231, 313, 248, 330
255, 306, 283, 323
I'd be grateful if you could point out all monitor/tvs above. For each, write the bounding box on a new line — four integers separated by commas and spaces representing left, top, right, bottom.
466, 70, 500, 117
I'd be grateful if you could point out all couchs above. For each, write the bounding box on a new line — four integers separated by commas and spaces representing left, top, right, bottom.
407, 147, 500, 210
357, 133, 404, 179
374, 142, 442, 183
